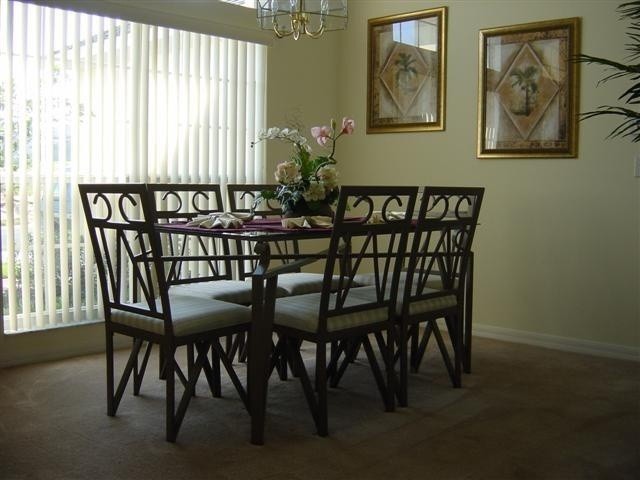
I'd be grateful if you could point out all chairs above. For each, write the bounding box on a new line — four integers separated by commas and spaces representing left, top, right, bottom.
335, 185, 485, 409
78, 184, 251, 443
353, 184, 477, 375
226, 183, 359, 383
149, 183, 284, 398
267, 184, 418, 442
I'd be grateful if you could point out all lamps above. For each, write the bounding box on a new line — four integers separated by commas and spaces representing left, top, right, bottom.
255, 0, 349, 41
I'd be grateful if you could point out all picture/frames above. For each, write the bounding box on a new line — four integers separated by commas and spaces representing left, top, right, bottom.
366, 5, 447, 134
475, 16, 583, 159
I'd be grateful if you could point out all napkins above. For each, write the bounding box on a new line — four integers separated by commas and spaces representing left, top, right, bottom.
366, 209, 406, 225
281, 215, 331, 228
183, 212, 252, 229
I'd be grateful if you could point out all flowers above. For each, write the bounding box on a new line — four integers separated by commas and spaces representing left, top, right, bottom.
249, 117, 355, 215
